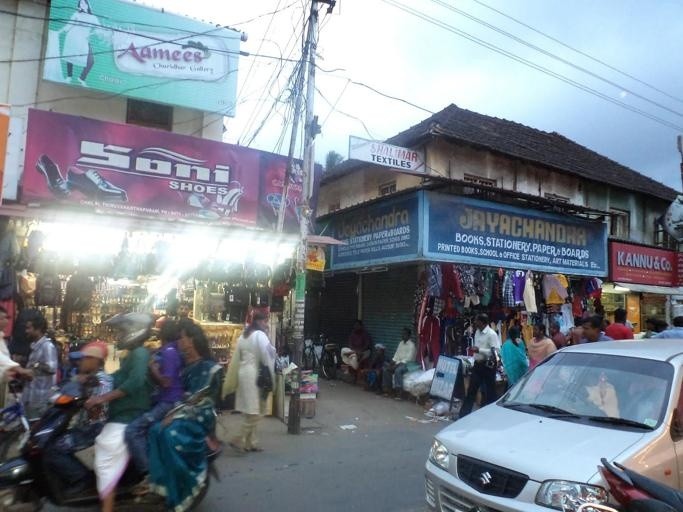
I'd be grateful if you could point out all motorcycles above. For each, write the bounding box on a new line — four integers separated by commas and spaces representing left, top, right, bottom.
578, 457, 683, 512
0, 377, 222, 511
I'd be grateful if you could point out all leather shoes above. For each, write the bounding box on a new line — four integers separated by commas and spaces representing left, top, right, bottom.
66, 164, 129, 205
35, 152, 72, 200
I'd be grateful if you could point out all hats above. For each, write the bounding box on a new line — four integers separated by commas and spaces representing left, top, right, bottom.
68, 340, 109, 362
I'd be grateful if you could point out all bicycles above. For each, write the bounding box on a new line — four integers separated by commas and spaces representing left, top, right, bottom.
303, 331, 360, 386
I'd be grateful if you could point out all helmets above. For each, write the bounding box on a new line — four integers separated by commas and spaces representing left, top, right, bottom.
106, 309, 155, 350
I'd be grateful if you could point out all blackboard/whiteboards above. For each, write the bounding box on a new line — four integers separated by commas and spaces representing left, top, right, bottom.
429, 354, 461, 401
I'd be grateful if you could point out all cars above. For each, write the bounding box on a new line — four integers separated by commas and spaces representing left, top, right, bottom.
422, 337, 682, 511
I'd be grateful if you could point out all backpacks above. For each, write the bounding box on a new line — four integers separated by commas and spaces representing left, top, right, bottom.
63, 275, 94, 311
34, 271, 63, 307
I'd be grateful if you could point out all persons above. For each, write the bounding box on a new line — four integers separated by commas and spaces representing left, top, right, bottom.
56, 1, 101, 85
381, 326, 416, 399
359, 343, 389, 395
500, 309, 683, 397
1, 300, 225, 511
349, 320, 371, 386
219, 312, 276, 456
451, 313, 499, 422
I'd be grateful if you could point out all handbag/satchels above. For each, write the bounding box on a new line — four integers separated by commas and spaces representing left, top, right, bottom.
257, 365, 273, 388
251, 284, 270, 308
224, 284, 251, 308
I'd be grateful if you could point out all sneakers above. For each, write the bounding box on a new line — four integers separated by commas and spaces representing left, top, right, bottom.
78, 77, 88, 88
64, 76, 72, 83
